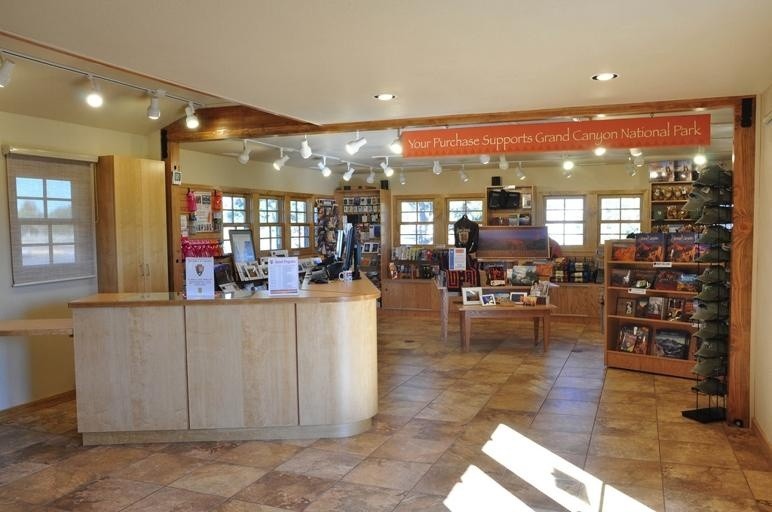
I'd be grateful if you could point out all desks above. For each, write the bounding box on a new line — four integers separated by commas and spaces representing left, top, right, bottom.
433, 277, 560, 338
454, 302, 553, 353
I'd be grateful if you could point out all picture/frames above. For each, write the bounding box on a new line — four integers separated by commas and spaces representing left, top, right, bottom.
474, 226, 550, 258
481, 294, 496, 306
463, 288, 482, 304
510, 292, 527, 304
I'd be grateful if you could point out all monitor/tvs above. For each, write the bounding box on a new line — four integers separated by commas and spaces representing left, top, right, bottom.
343, 223, 355, 273
334, 227, 344, 260
352, 230, 363, 282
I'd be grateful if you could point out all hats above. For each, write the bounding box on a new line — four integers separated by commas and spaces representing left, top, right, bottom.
692, 360, 727, 379
693, 285, 727, 302
694, 337, 727, 358
693, 164, 735, 186
697, 210, 731, 226
691, 381, 728, 397
695, 321, 728, 337
695, 226, 729, 247
690, 303, 728, 320
695, 266, 728, 284
694, 247, 731, 263
682, 186, 731, 211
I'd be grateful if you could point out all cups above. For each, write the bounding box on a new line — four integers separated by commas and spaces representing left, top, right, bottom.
338, 271, 352, 283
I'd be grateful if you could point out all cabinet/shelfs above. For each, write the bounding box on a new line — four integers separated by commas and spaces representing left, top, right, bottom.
335, 189, 391, 280
649, 181, 696, 233
95, 152, 169, 293
603, 239, 725, 383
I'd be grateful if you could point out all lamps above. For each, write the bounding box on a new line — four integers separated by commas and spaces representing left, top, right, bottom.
693, 150, 709, 166
85, 91, 104, 110
185, 101, 200, 129
345, 132, 367, 155
238, 140, 251, 165
273, 147, 290, 171
479, 154, 492, 165
366, 167, 375, 184
343, 162, 355, 182
433, 161, 442, 175
593, 146, 613, 159
459, 164, 469, 182
300, 134, 312, 159
317, 157, 332, 177
562, 152, 574, 170
380, 157, 394, 177
0, 52, 16, 87
623, 150, 637, 177
516, 161, 527, 181
146, 88, 166, 119
630, 148, 644, 168
399, 168, 406, 185
